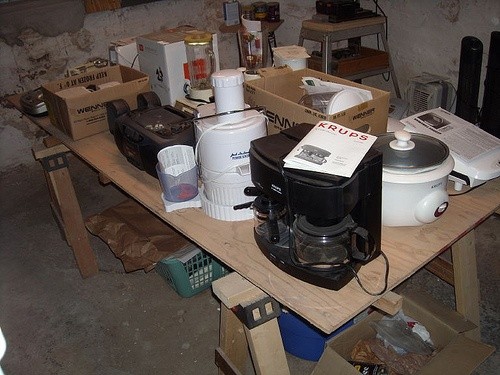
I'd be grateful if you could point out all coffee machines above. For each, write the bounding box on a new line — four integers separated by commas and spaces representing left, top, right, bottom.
234, 123, 378, 288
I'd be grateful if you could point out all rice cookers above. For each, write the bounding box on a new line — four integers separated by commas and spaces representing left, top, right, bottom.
371, 130, 455, 228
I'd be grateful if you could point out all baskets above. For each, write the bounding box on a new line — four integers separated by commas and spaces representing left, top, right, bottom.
155, 249, 230, 298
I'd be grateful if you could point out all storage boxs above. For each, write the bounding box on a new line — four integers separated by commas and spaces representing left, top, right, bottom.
41, 63, 150, 140
308, 291, 496, 375
137, 25, 219, 105
243, 68, 390, 133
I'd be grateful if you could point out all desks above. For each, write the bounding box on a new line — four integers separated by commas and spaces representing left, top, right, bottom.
8, 67, 499, 375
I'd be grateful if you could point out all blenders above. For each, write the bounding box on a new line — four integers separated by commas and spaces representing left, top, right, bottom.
193, 69, 266, 222
175, 30, 216, 118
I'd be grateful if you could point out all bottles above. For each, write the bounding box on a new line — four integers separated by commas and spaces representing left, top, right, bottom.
256, 2, 267, 19
244, 5, 255, 21
267, 2, 280, 23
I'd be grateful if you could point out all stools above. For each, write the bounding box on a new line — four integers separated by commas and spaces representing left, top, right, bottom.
299, 16, 402, 98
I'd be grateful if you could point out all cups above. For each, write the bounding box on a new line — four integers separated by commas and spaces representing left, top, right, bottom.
156, 145, 197, 202
